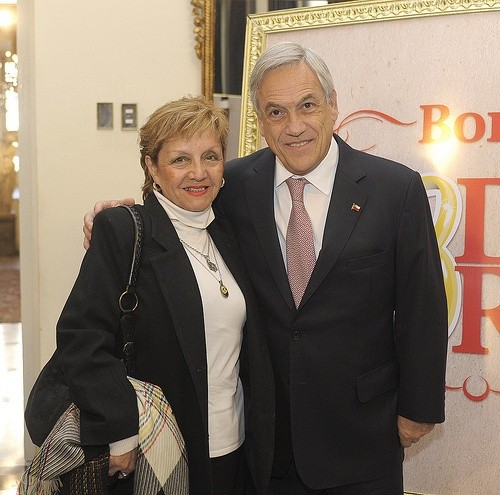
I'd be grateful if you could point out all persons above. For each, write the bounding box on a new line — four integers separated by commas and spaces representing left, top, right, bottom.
55, 97, 256, 495
81, 42, 448, 495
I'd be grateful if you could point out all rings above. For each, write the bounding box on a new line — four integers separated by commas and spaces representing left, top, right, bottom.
119, 470, 129, 476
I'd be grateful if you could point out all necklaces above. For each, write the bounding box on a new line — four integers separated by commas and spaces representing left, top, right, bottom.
182, 228, 229, 299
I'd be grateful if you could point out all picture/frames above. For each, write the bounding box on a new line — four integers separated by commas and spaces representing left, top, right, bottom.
239, 0, 500, 495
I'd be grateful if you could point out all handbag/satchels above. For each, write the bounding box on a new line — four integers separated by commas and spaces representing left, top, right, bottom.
23, 203, 145, 447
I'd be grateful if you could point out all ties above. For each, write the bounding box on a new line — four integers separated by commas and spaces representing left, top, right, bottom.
284, 178, 317, 308
